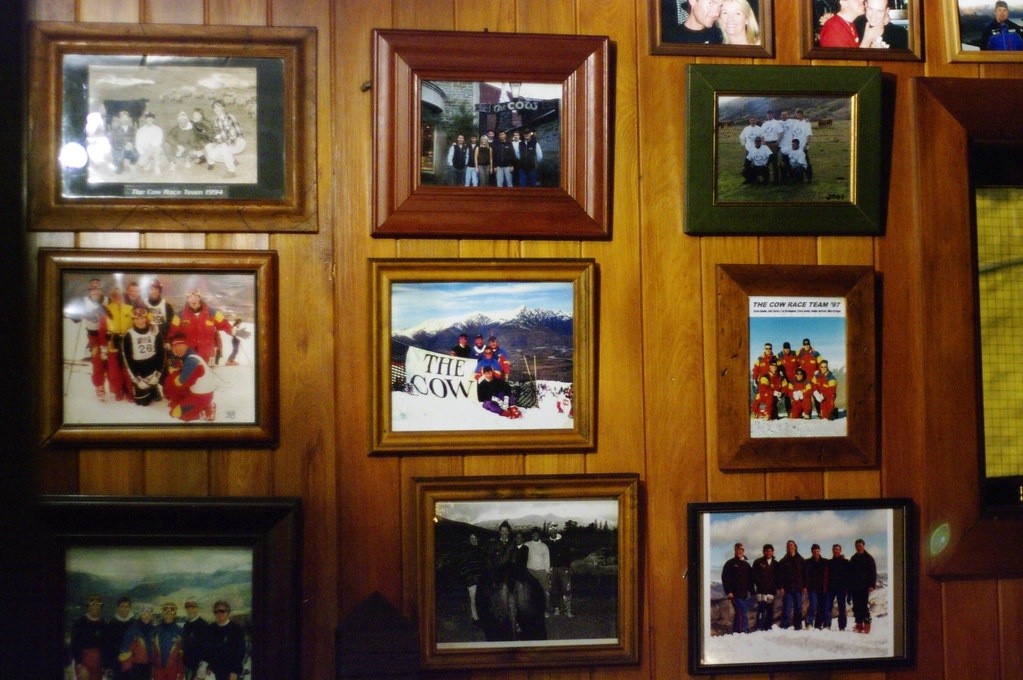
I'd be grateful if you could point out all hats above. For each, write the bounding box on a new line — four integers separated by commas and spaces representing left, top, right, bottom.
488, 336, 496, 341
460, 332, 468, 338
783, 342, 791, 349
803, 338, 810, 344
475, 333, 483, 339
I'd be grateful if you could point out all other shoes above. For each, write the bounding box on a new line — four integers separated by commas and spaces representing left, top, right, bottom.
126, 393, 134, 404
199, 403, 217, 420
227, 360, 238, 365
796, 623, 870, 634
501, 411, 515, 418
115, 390, 123, 401
509, 405, 521, 418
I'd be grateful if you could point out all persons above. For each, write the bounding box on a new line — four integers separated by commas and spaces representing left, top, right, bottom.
454, 523, 574, 641
450, 333, 520, 419
677, 0, 761, 46
979, 0, 1023, 51
447, 128, 543, 187
71, 596, 246, 680
750, 338, 837, 420
818, 0, 908, 50
740, 109, 813, 187
72, 278, 252, 421
720, 537, 876, 633
86, 99, 246, 183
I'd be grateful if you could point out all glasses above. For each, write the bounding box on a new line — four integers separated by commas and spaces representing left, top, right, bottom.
87, 597, 230, 620
484, 352, 492, 354
186, 288, 200, 296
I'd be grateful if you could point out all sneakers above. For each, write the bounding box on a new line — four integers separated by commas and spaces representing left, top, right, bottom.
96, 386, 105, 399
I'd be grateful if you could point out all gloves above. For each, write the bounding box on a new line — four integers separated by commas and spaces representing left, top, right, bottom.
145, 371, 162, 385
232, 326, 252, 340
131, 375, 150, 389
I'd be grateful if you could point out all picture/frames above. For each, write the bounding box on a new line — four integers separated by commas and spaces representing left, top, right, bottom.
715, 263, 879, 470
796, 0, 924, 63
366, 256, 597, 456
941, 1, 1023, 64
34, 247, 280, 449
680, 62, 884, 236
18, 494, 303, 680
686, 497, 915, 676
410, 471, 641, 671
648, 0, 776, 60
371, 29, 612, 241
28, 19, 321, 234
